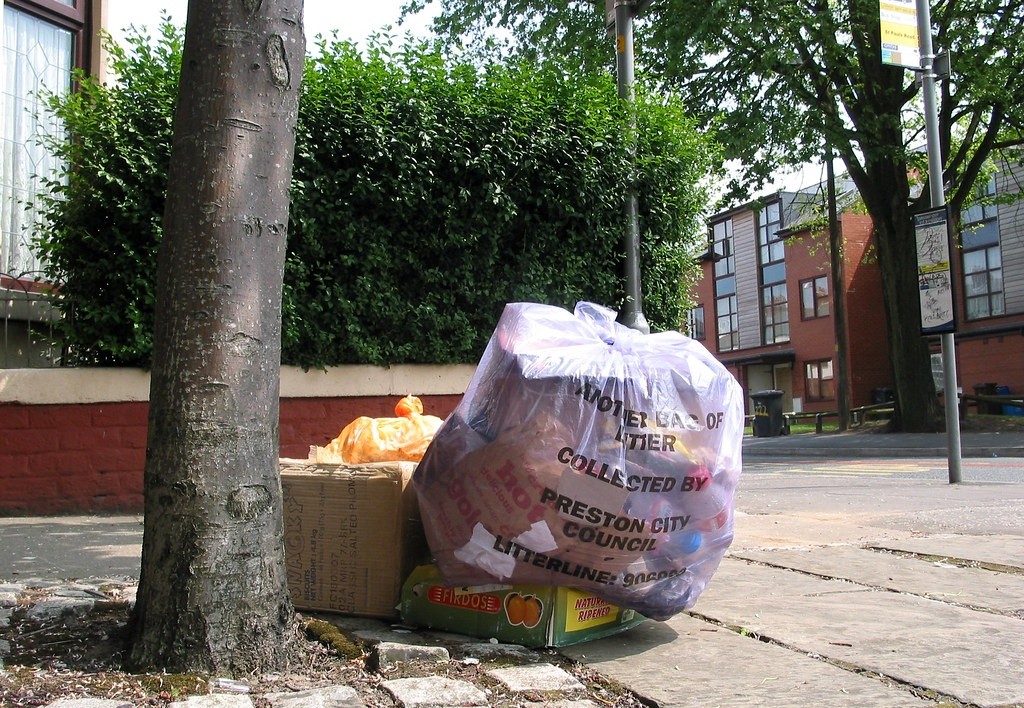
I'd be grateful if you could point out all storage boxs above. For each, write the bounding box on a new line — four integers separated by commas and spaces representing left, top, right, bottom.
401, 557, 648, 649
277, 458, 419, 619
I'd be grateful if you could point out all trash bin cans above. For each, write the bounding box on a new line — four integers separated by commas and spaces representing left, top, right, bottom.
748, 389, 785, 437
871, 387, 894, 420
973, 381, 1001, 414
